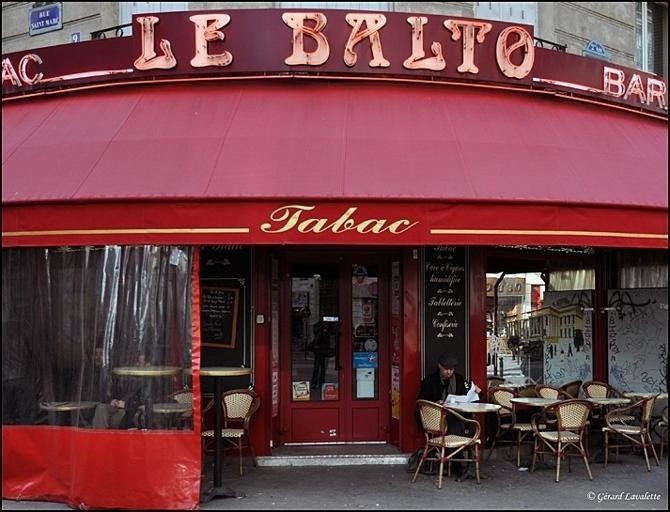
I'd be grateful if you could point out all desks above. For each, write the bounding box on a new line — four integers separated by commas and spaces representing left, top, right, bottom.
108, 366, 252, 505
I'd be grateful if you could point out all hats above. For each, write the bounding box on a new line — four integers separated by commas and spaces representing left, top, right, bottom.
439, 352, 460, 368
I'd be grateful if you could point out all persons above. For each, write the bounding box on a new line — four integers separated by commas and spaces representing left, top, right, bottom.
71, 334, 121, 427
505, 330, 585, 363
309, 304, 332, 390
1, 306, 45, 426
92, 348, 163, 428
414, 350, 483, 482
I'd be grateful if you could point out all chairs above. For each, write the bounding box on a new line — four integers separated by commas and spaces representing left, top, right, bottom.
411, 377, 668, 489
29, 366, 258, 475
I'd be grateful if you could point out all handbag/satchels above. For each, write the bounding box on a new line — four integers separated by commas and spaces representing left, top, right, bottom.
408, 447, 448, 473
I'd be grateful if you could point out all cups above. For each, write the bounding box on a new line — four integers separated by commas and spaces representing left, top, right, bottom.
449, 396, 456, 404
